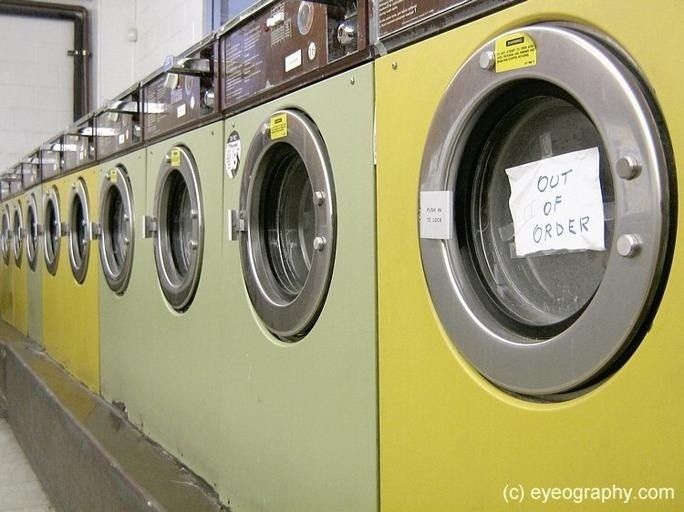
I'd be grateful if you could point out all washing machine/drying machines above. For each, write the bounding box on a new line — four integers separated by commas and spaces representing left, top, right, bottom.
0, 0, 684, 512
